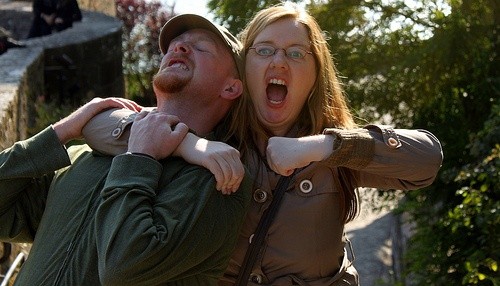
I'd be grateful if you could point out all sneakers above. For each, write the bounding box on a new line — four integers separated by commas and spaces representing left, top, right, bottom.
7, 11, 74, 45
0, 10, 34, 36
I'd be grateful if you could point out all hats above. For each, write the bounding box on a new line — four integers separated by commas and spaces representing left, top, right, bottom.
159, 14, 244, 81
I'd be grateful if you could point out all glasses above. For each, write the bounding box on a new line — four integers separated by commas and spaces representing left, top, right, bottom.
248, 45, 314, 59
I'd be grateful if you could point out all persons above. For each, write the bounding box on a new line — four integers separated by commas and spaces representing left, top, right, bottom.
81, 1, 443, 286
0, 14, 247, 286
